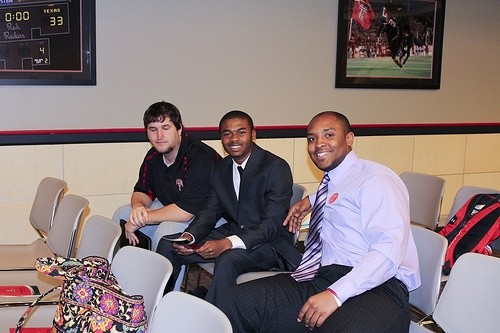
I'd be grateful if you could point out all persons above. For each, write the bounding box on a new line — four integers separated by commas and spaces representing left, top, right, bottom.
222, 112, 422, 333
112, 101, 224, 292
155, 110, 300, 310
391, 3, 422, 67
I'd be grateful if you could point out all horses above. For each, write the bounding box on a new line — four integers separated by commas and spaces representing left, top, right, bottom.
381, 15, 424, 69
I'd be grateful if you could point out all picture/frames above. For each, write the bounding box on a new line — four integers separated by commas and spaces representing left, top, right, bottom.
335, 0, 446, 89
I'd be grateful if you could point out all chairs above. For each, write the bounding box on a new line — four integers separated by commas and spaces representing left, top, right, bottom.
0, 172, 500, 333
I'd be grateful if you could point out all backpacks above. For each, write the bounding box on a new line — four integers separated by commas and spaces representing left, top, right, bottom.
435, 193, 500, 276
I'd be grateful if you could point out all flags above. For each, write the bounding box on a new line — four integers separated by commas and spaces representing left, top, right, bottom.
352, 0, 376, 32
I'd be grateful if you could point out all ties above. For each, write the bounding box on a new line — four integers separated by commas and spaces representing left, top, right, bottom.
237, 166, 244, 201
291, 174, 330, 282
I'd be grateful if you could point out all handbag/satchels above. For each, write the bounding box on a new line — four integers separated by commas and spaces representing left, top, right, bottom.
14, 256, 148, 333
